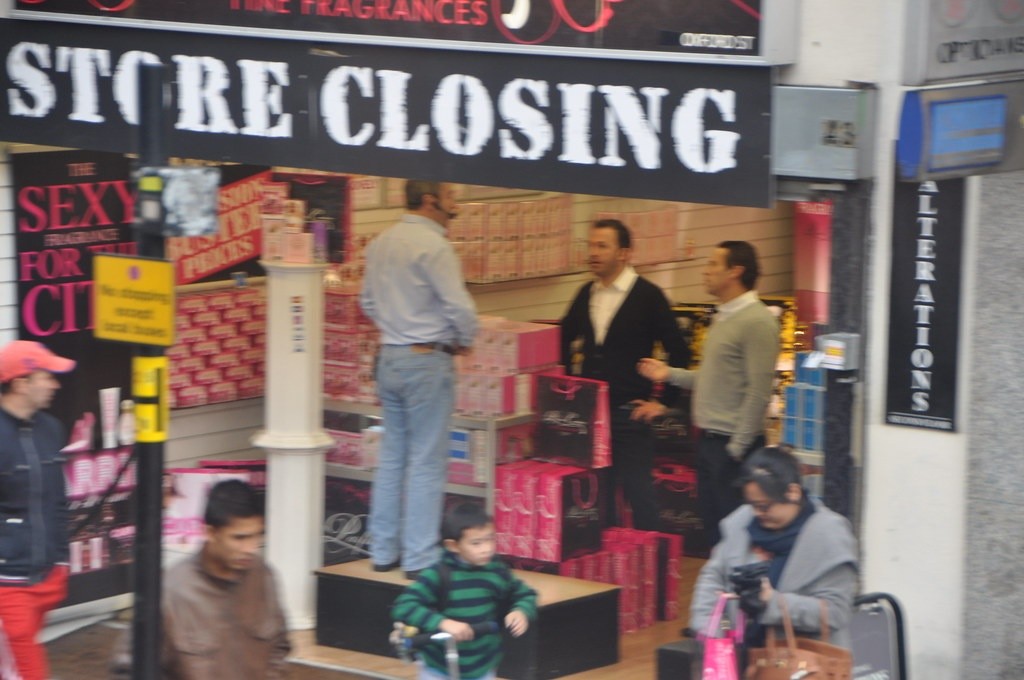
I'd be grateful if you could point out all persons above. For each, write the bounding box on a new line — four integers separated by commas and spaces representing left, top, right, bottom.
688, 445, 861, 680
1, 340, 76, 680
555, 219, 691, 532
392, 501, 539, 679
108, 479, 295, 680
638, 240, 782, 558
357, 178, 478, 580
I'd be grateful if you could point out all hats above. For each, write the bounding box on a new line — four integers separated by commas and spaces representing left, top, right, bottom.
0, 340, 79, 384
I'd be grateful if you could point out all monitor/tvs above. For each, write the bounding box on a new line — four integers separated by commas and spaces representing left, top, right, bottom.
898, 83, 1024, 184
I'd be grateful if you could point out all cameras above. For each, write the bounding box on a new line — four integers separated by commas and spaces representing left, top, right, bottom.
730, 561, 772, 620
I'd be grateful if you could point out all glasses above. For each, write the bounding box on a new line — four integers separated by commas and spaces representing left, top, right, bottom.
745, 498, 775, 514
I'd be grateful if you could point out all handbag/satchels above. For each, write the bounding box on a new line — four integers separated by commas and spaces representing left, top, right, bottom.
695, 592, 745, 680
492, 368, 715, 634
741, 591, 853, 680
